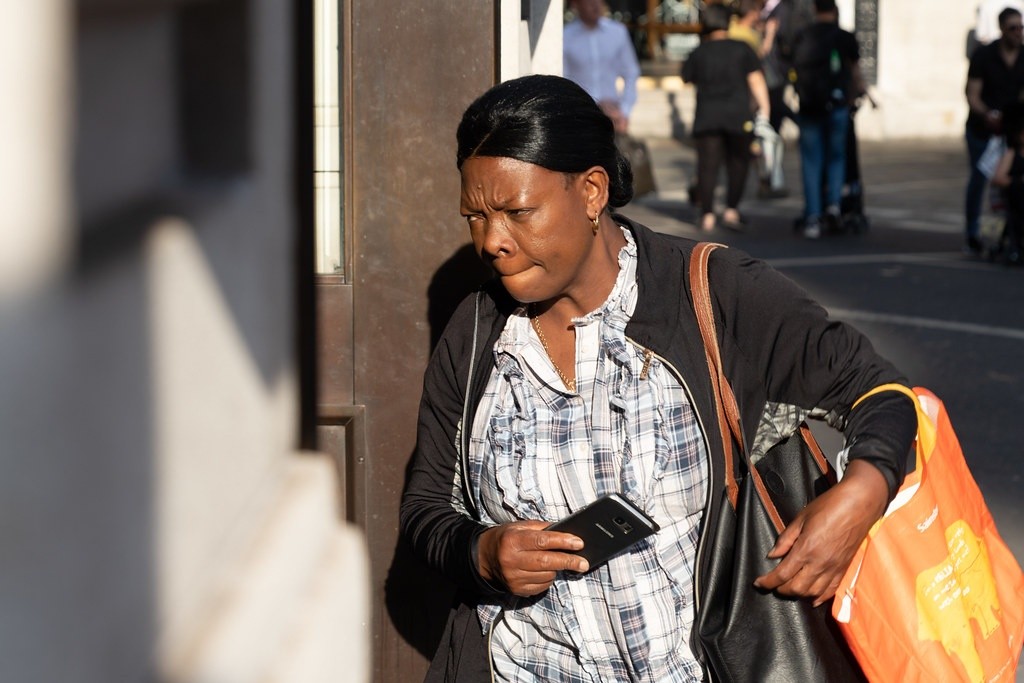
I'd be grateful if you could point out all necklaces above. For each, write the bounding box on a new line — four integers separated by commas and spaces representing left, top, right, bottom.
529, 303, 575, 390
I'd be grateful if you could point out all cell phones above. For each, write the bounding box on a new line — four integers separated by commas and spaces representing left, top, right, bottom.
542, 493, 655, 575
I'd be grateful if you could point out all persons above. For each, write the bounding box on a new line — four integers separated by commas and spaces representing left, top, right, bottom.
397, 74, 920, 682
563, 0, 1023, 265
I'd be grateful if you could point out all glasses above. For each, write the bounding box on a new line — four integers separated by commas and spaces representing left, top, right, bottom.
1005, 24, 1023, 32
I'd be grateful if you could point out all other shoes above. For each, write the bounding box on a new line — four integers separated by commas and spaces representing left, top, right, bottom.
793, 216, 819, 238
757, 178, 771, 198
962, 238, 983, 257
823, 217, 844, 233
697, 214, 717, 235
721, 209, 744, 231
763, 189, 788, 199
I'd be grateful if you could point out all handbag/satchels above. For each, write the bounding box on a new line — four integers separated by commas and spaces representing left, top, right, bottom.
690, 242, 869, 683
831, 386, 1024, 683
